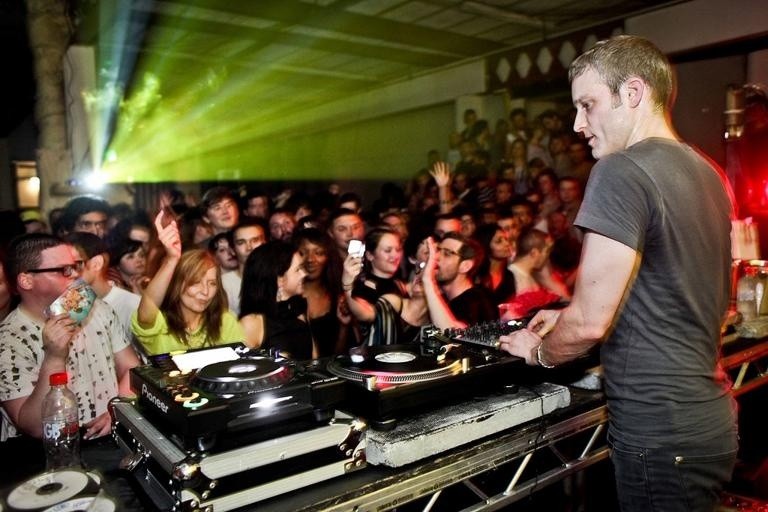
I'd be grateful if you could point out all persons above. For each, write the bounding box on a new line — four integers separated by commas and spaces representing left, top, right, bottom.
1, 107, 601, 318
727, 82, 768, 262
498, 34, 740, 511
132, 208, 249, 361
288, 226, 344, 360
495, 229, 572, 323
403, 229, 498, 344
62, 231, 148, 369
233, 238, 320, 366
342, 227, 408, 347
0, 231, 143, 444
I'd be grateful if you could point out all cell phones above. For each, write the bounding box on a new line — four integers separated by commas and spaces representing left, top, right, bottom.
348, 239, 366, 259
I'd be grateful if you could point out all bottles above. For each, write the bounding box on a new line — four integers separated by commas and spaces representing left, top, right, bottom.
41, 373, 85, 471
736, 260, 768, 339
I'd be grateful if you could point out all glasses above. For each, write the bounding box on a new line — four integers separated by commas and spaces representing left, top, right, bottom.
435, 247, 459, 257
28, 260, 83, 277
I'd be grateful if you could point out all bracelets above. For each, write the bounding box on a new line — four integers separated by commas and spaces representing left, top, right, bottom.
343, 284, 353, 291
536, 335, 558, 373
441, 199, 454, 204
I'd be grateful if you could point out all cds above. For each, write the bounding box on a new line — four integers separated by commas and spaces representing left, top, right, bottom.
7, 468, 88, 509
45, 497, 116, 512
